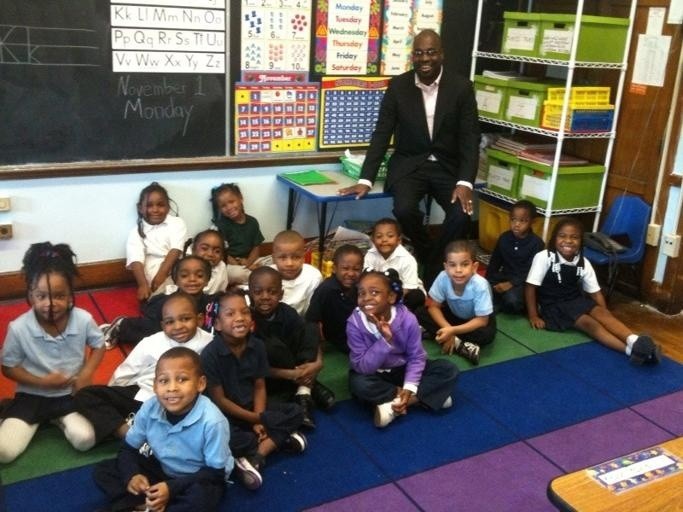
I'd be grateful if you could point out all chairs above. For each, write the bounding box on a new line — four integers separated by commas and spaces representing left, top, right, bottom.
580, 194, 653, 304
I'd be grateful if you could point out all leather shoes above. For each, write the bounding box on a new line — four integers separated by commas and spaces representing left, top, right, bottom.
644, 345, 662, 365
627, 335, 654, 364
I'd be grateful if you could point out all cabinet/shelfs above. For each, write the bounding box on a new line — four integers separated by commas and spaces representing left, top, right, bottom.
468, 0, 638, 270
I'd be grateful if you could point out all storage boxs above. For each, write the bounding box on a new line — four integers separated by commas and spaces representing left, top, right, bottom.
501, 11, 630, 64
474, 74, 580, 128
476, 195, 584, 255
484, 147, 606, 208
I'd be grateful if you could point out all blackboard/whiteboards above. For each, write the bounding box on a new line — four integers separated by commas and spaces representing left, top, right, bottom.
0, 0, 531, 180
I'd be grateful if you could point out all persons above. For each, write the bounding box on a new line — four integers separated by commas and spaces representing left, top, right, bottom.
77, 182, 335, 512
486, 201, 661, 364
337, 29, 480, 252
0, 239, 104, 465
305, 218, 497, 427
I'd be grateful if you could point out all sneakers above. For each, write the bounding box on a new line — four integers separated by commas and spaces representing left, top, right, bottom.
235, 453, 263, 490
103, 315, 125, 351
295, 394, 317, 428
375, 398, 400, 428
288, 431, 308, 452
128, 417, 154, 457
312, 380, 335, 411
457, 341, 480, 366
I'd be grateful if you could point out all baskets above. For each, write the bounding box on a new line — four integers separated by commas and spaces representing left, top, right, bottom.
311, 252, 333, 278
548, 86, 610, 108
339, 155, 389, 183
541, 99, 614, 132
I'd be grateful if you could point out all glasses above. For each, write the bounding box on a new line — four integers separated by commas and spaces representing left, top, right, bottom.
414, 50, 437, 56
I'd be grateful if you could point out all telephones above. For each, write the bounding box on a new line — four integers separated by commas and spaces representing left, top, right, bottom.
581, 230, 616, 259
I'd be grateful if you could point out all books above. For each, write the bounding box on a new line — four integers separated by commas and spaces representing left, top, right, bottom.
490, 131, 589, 165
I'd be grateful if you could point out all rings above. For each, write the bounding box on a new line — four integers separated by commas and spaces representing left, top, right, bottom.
467, 199, 473, 204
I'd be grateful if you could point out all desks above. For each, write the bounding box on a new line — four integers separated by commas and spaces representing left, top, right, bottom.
276, 169, 486, 273
